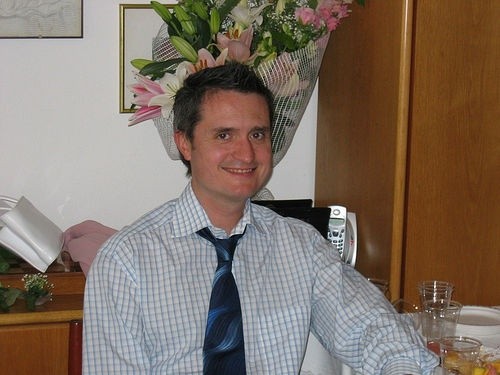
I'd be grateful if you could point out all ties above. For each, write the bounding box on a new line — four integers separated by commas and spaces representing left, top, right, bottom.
195, 224, 248, 375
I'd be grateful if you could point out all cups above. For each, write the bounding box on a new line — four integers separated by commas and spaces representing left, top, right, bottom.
391, 299, 421, 330
436, 336, 483, 375
416, 280, 455, 336
422, 298, 464, 356
366, 278, 388, 295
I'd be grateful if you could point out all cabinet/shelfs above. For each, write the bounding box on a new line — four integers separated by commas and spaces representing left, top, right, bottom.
0, 273, 86, 375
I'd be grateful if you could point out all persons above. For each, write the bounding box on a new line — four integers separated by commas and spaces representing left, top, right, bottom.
82, 63, 441, 375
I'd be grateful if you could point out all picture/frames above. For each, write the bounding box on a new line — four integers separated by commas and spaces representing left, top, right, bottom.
119, 4, 179, 114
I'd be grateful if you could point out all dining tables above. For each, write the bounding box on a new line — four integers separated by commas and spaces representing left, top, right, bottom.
342, 306, 500, 375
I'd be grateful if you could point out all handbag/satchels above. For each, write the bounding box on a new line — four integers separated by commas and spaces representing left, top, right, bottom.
0, 196, 64, 272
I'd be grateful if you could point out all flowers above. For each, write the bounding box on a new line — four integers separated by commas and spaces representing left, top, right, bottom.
128, 0, 366, 168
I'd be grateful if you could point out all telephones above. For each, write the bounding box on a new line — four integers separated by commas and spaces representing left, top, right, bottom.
327, 205, 357, 269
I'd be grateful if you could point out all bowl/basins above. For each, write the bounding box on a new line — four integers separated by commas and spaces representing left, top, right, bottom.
446, 305, 500, 348
